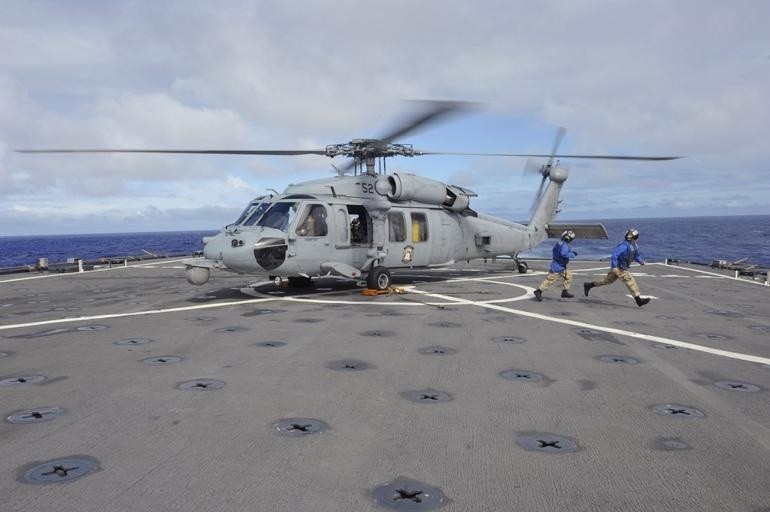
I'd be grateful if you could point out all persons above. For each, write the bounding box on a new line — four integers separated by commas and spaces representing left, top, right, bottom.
350, 217, 361, 241
533, 229, 579, 302
583, 227, 652, 308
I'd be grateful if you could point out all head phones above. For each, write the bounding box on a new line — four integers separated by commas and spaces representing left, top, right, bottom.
627, 232, 633, 240
564, 234, 569, 242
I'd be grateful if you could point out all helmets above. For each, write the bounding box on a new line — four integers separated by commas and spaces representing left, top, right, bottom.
562, 231, 575, 242
625, 229, 639, 241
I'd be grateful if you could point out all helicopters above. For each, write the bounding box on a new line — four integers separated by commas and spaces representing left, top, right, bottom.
7, 93, 686, 293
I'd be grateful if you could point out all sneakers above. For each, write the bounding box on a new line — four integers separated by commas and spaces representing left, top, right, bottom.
636, 298, 649, 306
561, 292, 573, 298
534, 290, 542, 301
584, 283, 592, 296
